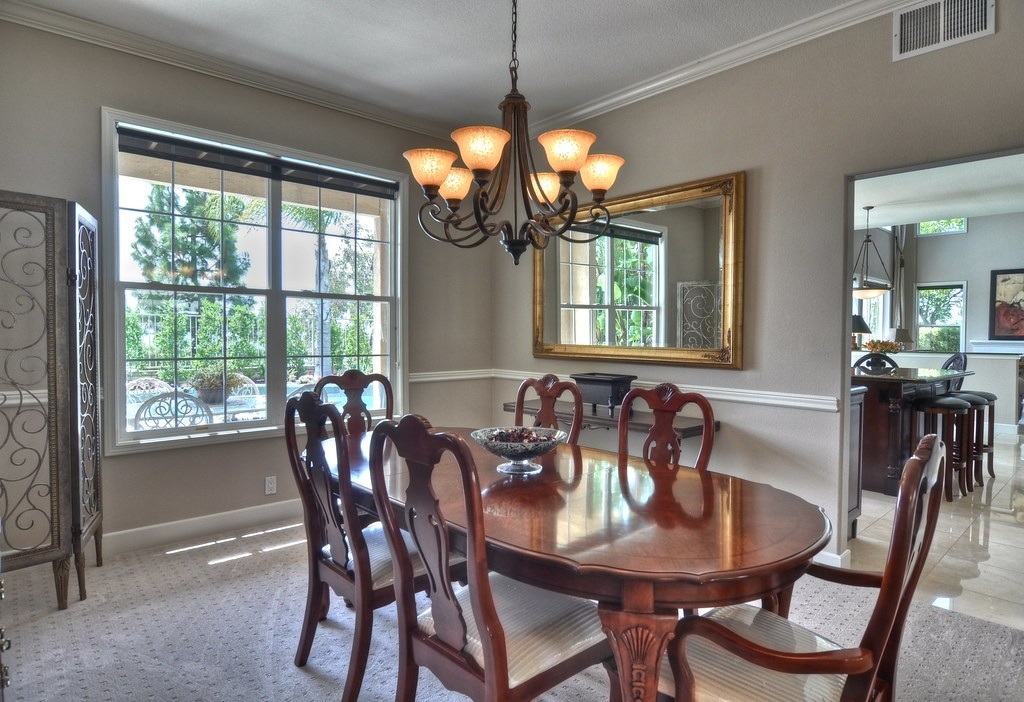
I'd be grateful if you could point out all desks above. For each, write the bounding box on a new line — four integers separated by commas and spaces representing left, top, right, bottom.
851, 367, 975, 498
503, 400, 719, 453
126, 394, 297, 432
300, 427, 833, 702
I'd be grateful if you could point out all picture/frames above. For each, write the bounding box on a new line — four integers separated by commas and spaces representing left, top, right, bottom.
988, 268, 1024, 341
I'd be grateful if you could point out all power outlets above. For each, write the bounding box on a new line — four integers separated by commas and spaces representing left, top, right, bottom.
265, 476, 277, 496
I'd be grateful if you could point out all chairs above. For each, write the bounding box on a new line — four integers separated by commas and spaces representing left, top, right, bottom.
126, 378, 174, 403
284, 390, 491, 702
369, 412, 622, 702
853, 353, 899, 367
514, 374, 582, 445
314, 369, 433, 608
134, 392, 213, 431
287, 384, 329, 418
618, 382, 715, 470
941, 353, 967, 391
602, 433, 948, 702
227, 373, 260, 396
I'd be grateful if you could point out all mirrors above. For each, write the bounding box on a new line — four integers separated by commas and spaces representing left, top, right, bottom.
533, 170, 746, 371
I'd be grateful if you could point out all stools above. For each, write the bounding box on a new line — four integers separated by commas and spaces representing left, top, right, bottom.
953, 390, 998, 480
910, 395, 971, 502
940, 392, 989, 492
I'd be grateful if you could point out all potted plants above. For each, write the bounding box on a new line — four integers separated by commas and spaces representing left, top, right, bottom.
188, 370, 246, 404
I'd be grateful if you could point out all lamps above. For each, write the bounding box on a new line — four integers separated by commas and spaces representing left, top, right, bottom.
852, 314, 873, 351
883, 325, 914, 352
852, 206, 896, 300
401, 0, 626, 268
